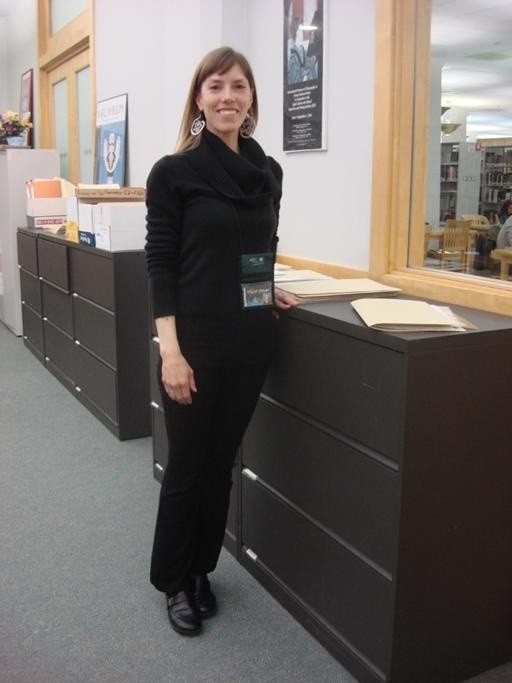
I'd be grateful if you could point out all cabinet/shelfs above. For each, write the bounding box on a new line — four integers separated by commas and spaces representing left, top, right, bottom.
439, 141, 512, 222
1, 147, 61, 336
15, 225, 152, 443
153, 287, 511, 682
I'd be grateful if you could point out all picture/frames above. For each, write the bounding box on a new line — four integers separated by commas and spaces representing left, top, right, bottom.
93, 92, 129, 188
281, 0, 329, 154
20, 69, 34, 148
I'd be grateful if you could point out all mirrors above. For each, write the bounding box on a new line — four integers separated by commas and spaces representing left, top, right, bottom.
370, 0, 512, 319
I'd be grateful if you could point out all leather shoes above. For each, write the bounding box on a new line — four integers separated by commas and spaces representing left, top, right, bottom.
189, 573, 217, 618
166, 585, 202, 636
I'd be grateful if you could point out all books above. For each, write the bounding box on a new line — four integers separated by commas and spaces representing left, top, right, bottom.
440, 148, 512, 224
43, 223, 66, 235
274, 262, 479, 332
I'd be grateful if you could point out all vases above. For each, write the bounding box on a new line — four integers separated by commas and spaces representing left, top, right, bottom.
5, 134, 26, 147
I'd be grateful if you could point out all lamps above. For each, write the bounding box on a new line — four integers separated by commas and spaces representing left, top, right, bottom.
440, 123, 461, 135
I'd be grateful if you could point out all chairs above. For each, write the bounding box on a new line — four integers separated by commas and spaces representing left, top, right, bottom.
424, 212, 511, 281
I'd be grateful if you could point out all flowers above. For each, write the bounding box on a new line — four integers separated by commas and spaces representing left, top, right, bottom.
0, 111, 34, 140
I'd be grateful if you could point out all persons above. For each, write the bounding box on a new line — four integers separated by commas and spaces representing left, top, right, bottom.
495, 216, 512, 276
284, 17, 321, 83
143, 43, 305, 641
499, 197, 511, 224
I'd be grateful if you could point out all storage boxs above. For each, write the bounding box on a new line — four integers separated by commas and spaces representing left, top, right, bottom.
21, 174, 148, 254
